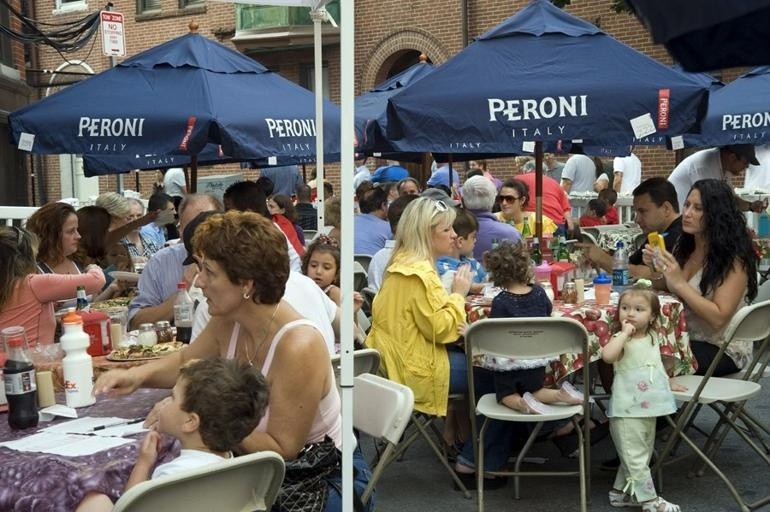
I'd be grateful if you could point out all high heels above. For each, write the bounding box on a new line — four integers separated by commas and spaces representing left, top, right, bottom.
439, 438, 508, 490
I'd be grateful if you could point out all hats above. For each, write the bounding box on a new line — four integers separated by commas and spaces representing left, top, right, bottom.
729, 141, 760, 167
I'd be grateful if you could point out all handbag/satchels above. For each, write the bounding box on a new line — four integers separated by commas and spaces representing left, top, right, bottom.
272, 433, 365, 512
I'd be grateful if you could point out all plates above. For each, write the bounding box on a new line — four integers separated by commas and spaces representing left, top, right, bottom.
108, 270, 140, 283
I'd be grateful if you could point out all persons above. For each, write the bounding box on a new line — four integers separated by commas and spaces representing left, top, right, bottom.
602, 283, 682, 512
1, 143, 769, 492
74, 356, 270, 511
92, 210, 375, 512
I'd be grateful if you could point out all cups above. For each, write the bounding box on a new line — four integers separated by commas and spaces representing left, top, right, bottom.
2, 325, 34, 371
108, 308, 128, 351
35, 371, 56, 408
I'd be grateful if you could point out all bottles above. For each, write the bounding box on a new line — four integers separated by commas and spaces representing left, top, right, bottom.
75, 285, 90, 313
563, 282, 577, 303
174, 283, 194, 344
0, 370, 8, 412
58, 311, 97, 408
593, 272, 613, 305
491, 238, 500, 250
524, 234, 571, 265
613, 242, 629, 295
575, 277, 586, 304
139, 319, 175, 347
3, 337, 39, 429
540, 280, 554, 305
521, 217, 531, 239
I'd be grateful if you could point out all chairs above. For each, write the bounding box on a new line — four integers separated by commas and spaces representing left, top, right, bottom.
112, 447, 287, 512
349, 375, 420, 510
357, 313, 472, 502
690, 276, 770, 478
651, 300, 769, 512
460, 315, 598, 512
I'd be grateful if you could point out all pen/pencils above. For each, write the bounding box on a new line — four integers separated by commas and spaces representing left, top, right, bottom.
94, 422, 125, 430
68, 433, 97, 436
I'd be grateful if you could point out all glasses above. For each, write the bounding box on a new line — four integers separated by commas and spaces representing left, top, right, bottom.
495, 195, 521, 204
431, 200, 449, 218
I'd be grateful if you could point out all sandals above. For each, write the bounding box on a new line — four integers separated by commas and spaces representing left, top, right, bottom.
642, 496, 681, 512
559, 380, 595, 405
520, 392, 555, 415
608, 488, 642, 507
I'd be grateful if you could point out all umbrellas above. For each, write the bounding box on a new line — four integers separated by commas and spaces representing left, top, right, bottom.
352, 53, 437, 152
672, 64, 770, 149
386, 0, 710, 240
7, 21, 358, 194
